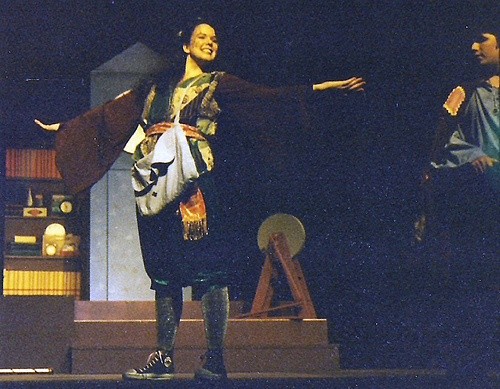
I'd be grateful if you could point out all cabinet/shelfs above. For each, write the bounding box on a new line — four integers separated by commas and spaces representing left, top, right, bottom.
3, 149, 82, 298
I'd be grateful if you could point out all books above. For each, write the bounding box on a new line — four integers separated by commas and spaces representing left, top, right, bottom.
3, 147, 82, 298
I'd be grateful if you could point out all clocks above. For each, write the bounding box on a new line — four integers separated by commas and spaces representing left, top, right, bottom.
60, 200, 72, 213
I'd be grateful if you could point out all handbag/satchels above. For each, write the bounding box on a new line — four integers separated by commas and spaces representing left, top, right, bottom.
130, 121, 200, 220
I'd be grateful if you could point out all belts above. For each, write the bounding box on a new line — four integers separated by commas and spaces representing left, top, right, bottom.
142, 123, 207, 140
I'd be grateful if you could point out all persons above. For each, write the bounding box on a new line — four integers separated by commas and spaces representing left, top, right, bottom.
32, 17, 371, 381
424, 11, 499, 387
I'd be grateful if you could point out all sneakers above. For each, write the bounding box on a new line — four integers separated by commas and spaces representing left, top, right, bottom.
122, 349, 174, 380
195, 350, 227, 381
